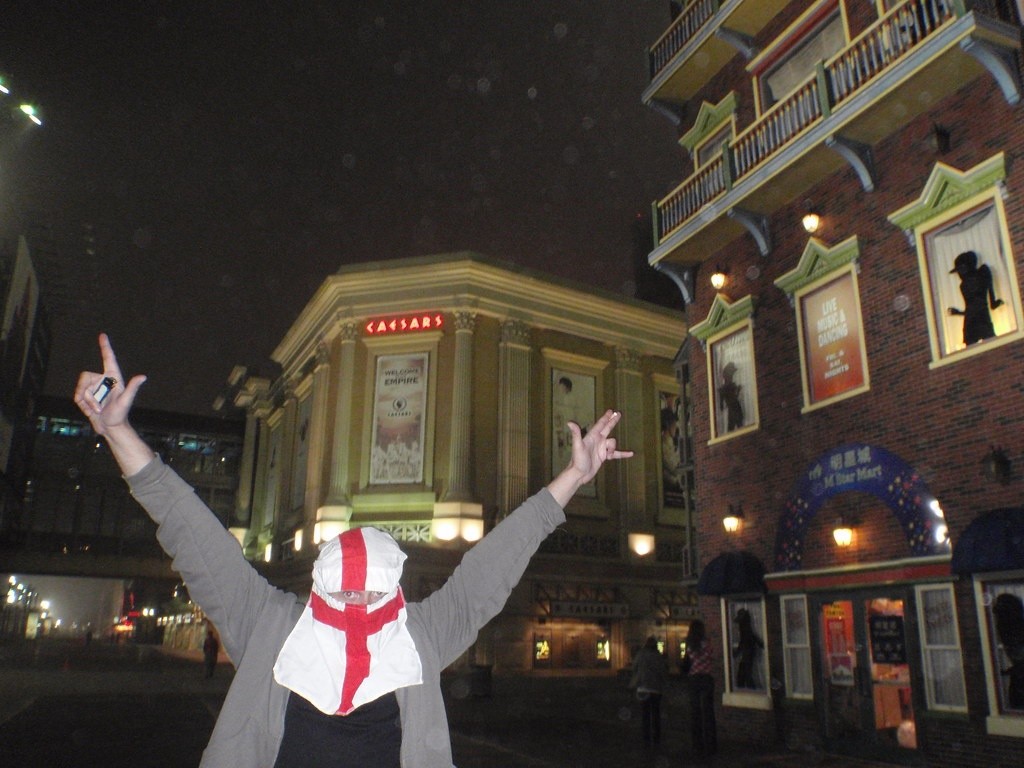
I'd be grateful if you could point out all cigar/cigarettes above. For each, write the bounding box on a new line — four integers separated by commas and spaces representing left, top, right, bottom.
609, 412, 618, 421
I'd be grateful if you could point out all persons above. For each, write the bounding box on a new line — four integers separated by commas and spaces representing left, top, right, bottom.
681, 619, 719, 757
86, 629, 93, 646
661, 408, 691, 495
203, 631, 219, 680
558, 376, 596, 488
75, 332, 634, 768
634, 637, 671, 751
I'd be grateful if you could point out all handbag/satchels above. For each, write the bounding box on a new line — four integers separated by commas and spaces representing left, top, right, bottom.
675, 651, 690, 668
646, 671, 674, 690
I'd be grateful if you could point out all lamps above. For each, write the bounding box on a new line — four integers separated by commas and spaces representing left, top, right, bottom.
723, 505, 739, 533
803, 208, 821, 235
710, 262, 731, 290
832, 514, 853, 549
923, 121, 950, 156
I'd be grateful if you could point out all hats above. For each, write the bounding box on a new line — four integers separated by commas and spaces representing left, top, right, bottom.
722, 362, 738, 375
949, 251, 976, 273
733, 609, 751, 623
660, 408, 678, 428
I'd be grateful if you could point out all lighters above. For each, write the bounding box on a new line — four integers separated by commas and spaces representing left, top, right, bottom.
92, 377, 117, 403
